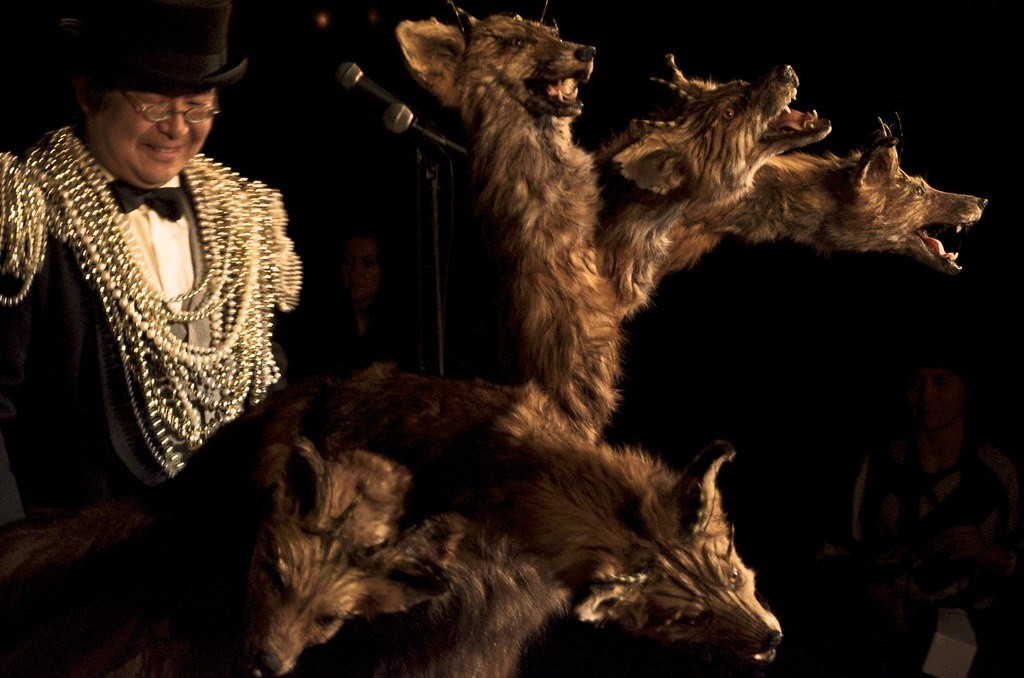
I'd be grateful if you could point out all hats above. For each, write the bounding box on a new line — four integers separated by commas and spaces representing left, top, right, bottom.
60, 0, 248, 90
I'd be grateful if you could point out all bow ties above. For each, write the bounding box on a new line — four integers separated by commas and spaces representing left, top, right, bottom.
110, 177, 183, 222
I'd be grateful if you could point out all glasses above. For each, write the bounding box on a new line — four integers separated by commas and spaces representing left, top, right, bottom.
121, 91, 222, 125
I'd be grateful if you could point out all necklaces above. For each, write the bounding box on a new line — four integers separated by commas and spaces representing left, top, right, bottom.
40, 115, 281, 479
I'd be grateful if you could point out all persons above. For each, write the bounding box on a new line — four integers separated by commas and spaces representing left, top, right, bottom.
0, 0, 306, 549
815, 332, 1023, 678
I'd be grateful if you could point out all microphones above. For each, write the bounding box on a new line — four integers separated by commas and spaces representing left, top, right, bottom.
383, 102, 466, 156
334, 61, 398, 107
